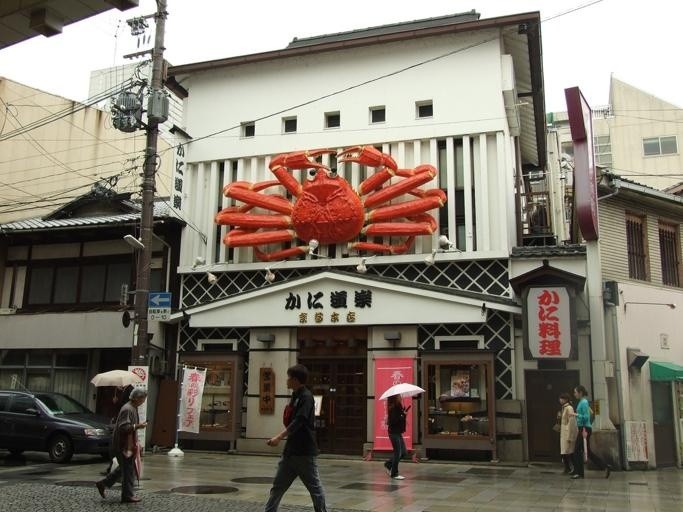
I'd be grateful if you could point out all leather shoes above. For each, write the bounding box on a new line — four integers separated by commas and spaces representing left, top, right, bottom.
606, 464, 612, 478
562, 469, 584, 479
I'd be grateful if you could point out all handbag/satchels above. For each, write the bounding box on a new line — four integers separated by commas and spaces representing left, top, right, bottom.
110, 456, 123, 483
583, 433, 587, 463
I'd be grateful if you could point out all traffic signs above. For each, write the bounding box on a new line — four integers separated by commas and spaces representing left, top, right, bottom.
149, 293, 171, 321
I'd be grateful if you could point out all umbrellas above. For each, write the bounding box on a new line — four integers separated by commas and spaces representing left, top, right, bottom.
378, 383, 426, 409
90, 370, 144, 398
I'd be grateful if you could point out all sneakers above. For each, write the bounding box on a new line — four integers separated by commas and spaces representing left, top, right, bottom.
96, 481, 105, 498
384, 464, 406, 480
121, 494, 142, 501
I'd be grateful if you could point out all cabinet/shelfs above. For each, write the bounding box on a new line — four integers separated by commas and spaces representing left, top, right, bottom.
177, 351, 244, 455
421, 350, 499, 462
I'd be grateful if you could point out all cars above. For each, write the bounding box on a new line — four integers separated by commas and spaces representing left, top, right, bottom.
0, 388, 115, 464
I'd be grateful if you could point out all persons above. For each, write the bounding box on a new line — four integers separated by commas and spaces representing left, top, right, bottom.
265, 364, 327, 512
557, 392, 578, 475
96, 388, 148, 503
384, 394, 409, 480
101, 384, 135, 475
568, 385, 612, 479
